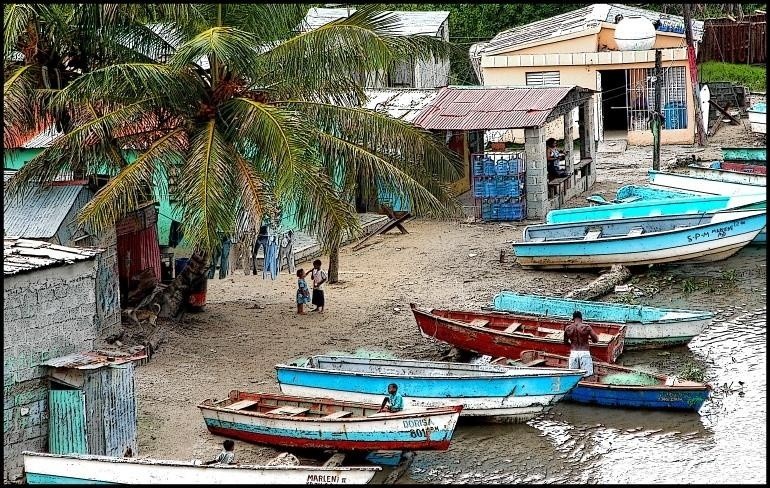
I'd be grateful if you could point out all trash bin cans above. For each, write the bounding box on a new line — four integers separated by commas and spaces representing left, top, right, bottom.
159, 252, 174, 285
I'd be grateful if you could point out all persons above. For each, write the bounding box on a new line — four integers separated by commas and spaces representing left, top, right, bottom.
563, 311, 598, 382
310, 260, 326, 314
375, 383, 403, 413
295, 268, 315, 315
546, 137, 567, 198
200, 439, 240, 464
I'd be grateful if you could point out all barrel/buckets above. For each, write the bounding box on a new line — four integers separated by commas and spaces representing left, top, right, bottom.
187, 277, 207, 313
472, 155, 527, 220
663, 103, 687, 129
175, 258, 190, 280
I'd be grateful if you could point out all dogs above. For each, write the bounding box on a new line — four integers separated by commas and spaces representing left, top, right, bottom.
121, 302, 162, 329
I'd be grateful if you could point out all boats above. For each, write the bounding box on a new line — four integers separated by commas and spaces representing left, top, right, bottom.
196, 389, 463, 455
492, 291, 716, 352
408, 302, 626, 364
487, 350, 710, 416
24, 451, 384, 484
275, 356, 587, 426
